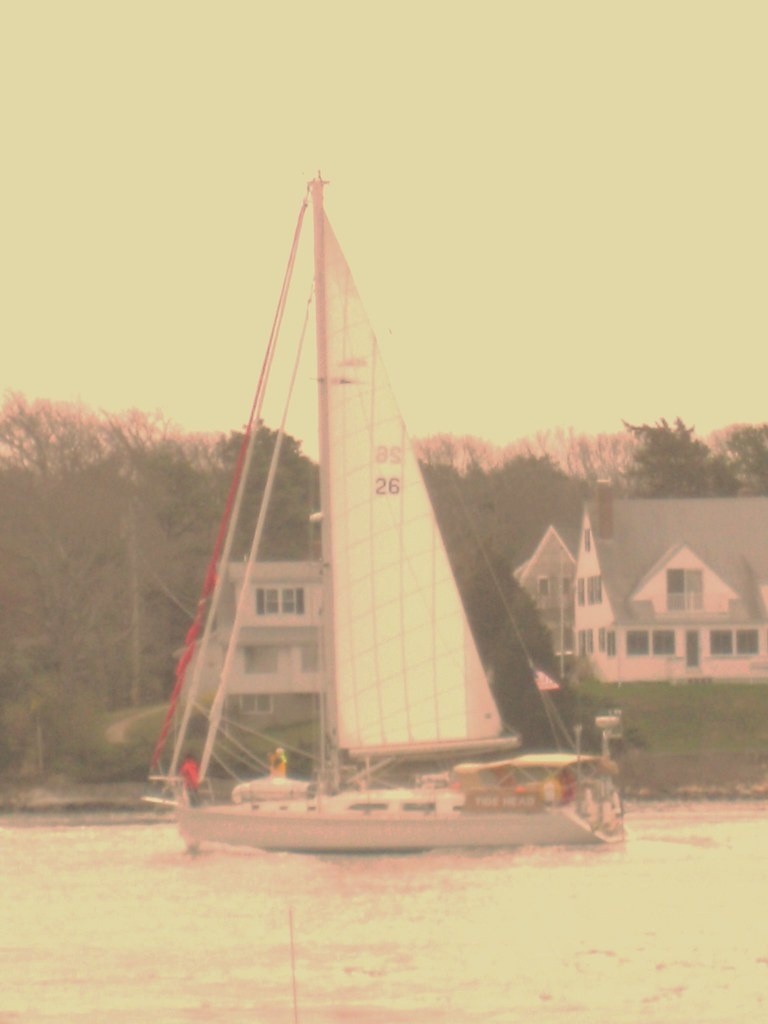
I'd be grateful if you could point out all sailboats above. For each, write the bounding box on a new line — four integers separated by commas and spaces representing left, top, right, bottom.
135, 173, 629, 851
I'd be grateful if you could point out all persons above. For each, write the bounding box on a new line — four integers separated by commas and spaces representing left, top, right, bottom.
180, 753, 198, 808
267, 747, 286, 779
